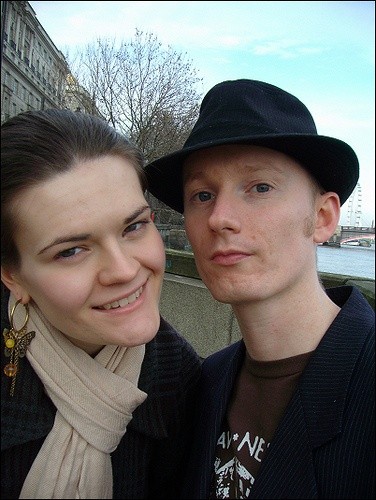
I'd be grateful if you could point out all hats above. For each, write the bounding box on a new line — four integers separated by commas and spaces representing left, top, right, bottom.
142, 79, 359, 214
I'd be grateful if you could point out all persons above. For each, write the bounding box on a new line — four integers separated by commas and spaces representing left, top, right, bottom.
0, 108, 203, 500
138, 79, 376, 500
